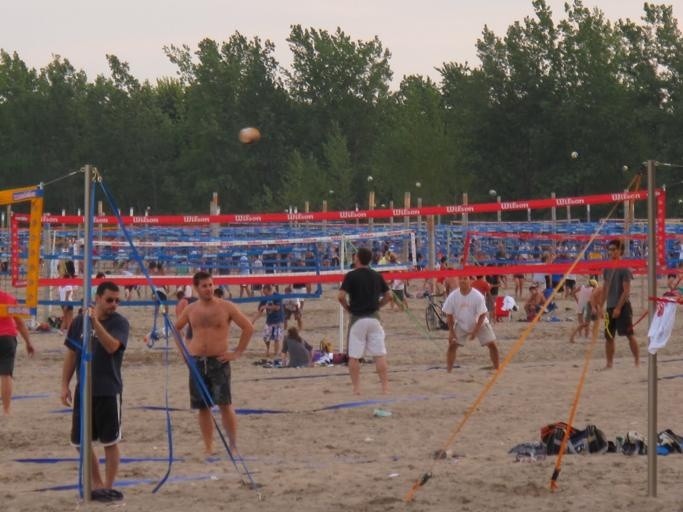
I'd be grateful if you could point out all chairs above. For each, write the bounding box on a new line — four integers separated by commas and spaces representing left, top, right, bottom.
495, 296, 510, 321
536, 301, 556, 322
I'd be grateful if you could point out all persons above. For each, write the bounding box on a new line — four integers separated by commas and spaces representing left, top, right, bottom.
173, 272, 254, 457
59, 282, 129, 501
0, 235, 682, 414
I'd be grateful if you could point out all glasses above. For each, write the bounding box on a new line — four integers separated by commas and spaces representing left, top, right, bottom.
101, 295, 121, 304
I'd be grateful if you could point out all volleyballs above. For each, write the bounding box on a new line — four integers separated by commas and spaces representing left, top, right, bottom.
238, 127, 259, 145
